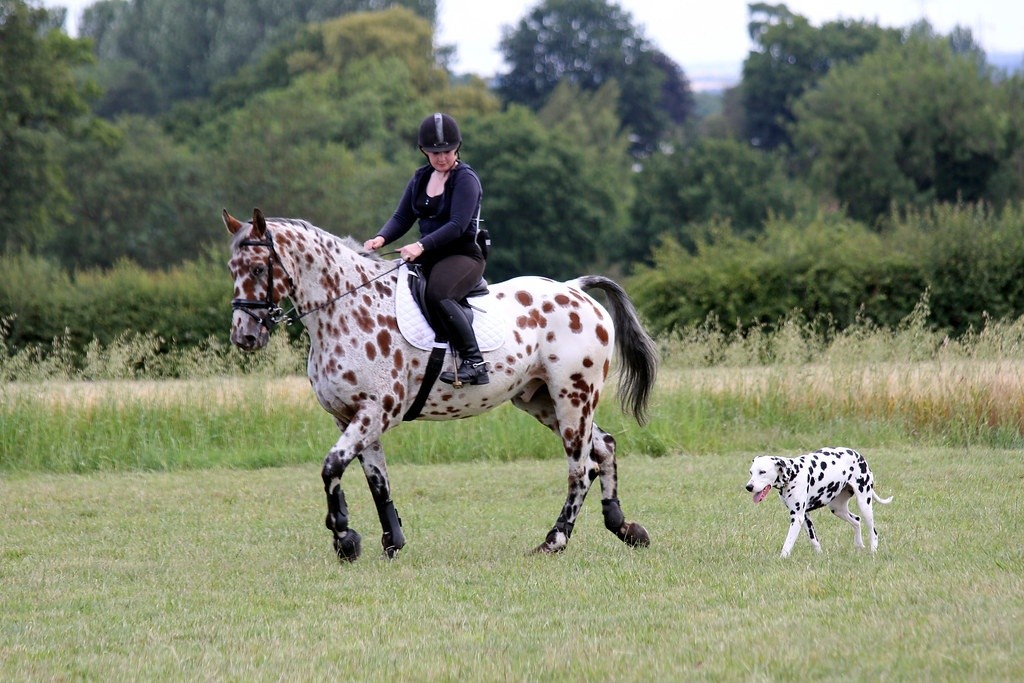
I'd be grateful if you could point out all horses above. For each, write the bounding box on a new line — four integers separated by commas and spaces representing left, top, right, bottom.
220, 206, 661, 562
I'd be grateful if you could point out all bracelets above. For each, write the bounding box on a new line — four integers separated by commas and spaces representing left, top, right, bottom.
417, 241, 425, 251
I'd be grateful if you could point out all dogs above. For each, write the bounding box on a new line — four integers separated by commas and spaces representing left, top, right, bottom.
745, 447, 894, 560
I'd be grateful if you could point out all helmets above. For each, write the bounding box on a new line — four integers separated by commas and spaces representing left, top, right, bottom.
417, 112, 463, 154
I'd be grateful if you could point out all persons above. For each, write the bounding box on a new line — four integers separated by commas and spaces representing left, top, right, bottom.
363, 113, 492, 386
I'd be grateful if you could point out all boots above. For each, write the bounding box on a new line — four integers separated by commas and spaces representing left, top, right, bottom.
433, 298, 490, 385
